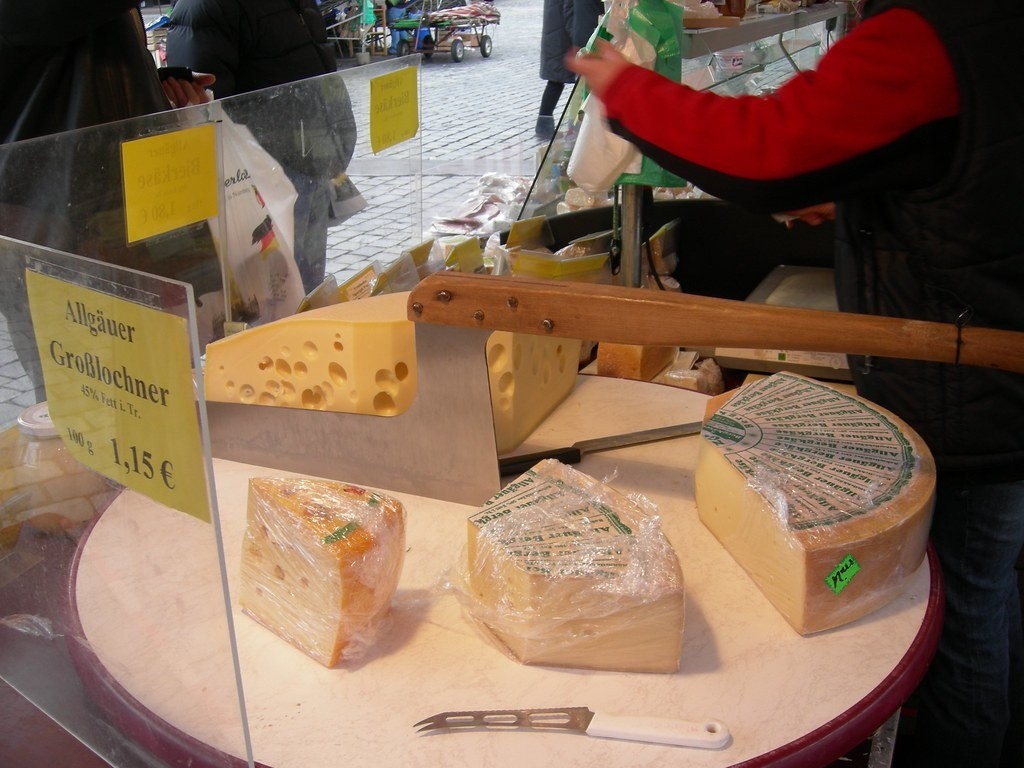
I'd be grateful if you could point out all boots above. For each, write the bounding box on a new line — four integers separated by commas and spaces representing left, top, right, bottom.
536, 84, 565, 139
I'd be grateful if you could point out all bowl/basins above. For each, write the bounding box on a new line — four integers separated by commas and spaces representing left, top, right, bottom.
714, 51, 746, 70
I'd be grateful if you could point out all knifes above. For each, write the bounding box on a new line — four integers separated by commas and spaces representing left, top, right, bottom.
498, 421, 703, 476
412, 707, 729, 749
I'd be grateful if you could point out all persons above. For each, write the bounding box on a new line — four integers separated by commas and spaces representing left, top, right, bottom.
385, 0, 409, 56
0, 0, 224, 408
566, 0, 1024, 768
535, 0, 605, 139
165, 0, 357, 298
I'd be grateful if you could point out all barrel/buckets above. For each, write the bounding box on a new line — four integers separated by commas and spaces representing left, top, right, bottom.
414, 28, 430, 50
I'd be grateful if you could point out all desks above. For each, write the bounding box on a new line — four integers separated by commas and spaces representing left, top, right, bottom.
67, 374, 944, 768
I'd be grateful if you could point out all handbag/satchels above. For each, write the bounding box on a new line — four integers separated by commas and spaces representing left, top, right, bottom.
170, 89, 306, 329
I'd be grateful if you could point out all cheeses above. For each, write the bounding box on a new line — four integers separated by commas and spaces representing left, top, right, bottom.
203, 236, 936, 676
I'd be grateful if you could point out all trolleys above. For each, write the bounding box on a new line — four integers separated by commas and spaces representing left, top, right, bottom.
389, 21, 500, 63
